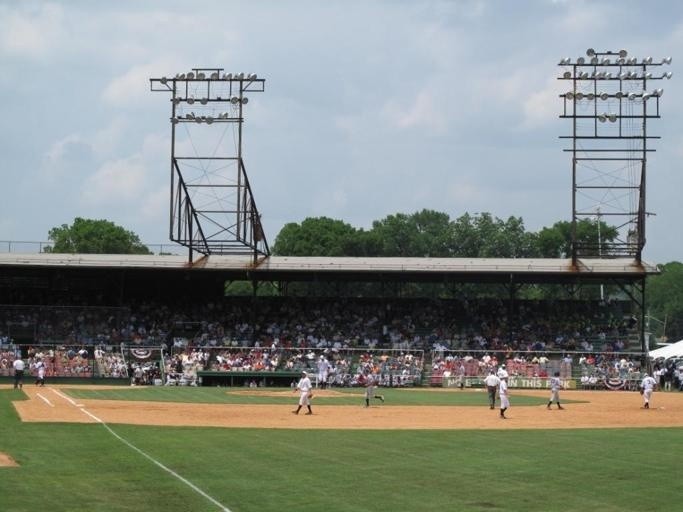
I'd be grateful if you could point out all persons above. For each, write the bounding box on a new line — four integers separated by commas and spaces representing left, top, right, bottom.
358, 368, 384, 408
2, 285, 652, 389
545, 371, 566, 411
483, 370, 501, 410
291, 370, 315, 416
498, 375, 511, 420
639, 371, 658, 409
650, 356, 676, 391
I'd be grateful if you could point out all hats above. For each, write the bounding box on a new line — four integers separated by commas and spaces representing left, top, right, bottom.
301, 371, 308, 375
643, 373, 649, 377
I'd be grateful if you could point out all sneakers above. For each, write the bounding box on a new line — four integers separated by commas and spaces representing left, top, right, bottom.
488, 403, 652, 420
291, 395, 385, 417
